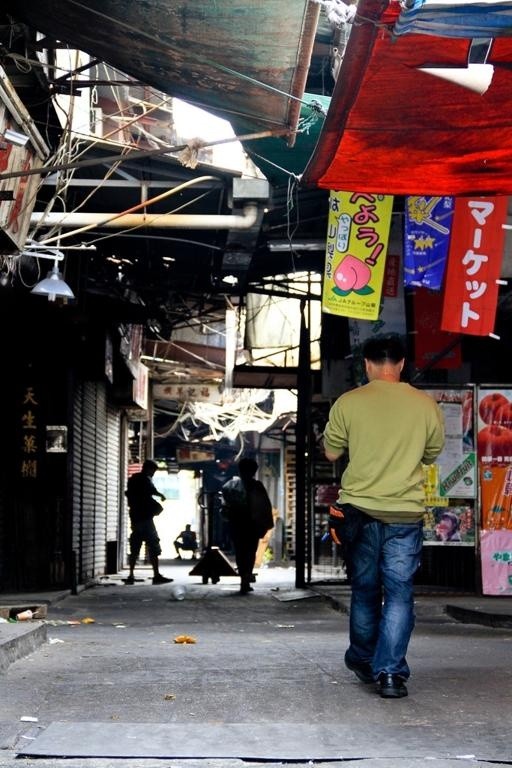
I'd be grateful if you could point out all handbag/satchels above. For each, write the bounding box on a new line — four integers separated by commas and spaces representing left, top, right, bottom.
328, 505, 365, 546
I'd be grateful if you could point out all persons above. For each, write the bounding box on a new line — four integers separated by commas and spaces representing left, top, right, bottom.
173, 525, 199, 560
328, 515, 347, 571
436, 512, 461, 541
322, 331, 447, 698
218, 458, 275, 594
124, 459, 174, 585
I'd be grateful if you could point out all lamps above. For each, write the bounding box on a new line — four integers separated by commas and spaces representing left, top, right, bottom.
28, 226, 74, 303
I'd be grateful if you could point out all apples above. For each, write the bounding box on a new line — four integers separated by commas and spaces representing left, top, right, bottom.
477, 425, 512, 462
479, 391, 508, 422
494, 400, 512, 422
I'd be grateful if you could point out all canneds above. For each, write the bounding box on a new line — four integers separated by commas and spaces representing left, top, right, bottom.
423, 462, 440, 498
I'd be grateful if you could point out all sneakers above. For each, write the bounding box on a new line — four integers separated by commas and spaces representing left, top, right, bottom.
153, 575, 173, 584
345, 650, 408, 698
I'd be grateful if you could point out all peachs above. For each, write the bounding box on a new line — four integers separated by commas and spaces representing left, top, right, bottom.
331, 254, 376, 297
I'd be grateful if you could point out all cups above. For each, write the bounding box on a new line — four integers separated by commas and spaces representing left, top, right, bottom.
171, 585, 186, 599
16, 609, 35, 621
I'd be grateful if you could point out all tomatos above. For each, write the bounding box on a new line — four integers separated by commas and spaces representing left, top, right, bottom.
487, 504, 509, 525
